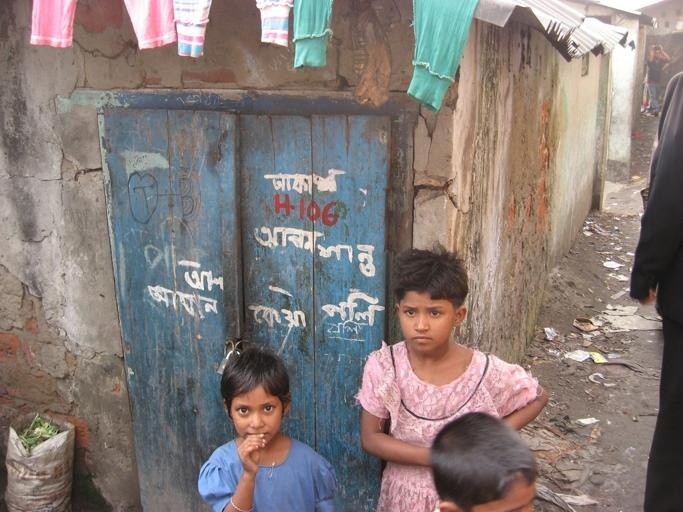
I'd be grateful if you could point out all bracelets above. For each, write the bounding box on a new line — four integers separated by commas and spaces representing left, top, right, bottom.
230, 496, 255, 512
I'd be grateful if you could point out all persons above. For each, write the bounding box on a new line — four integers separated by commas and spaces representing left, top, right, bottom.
357, 242, 550, 512
629, 70, 683, 512
197, 347, 338, 512
428, 410, 539, 512
645, 43, 672, 116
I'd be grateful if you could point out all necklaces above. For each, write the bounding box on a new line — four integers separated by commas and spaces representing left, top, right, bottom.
262, 453, 278, 479
